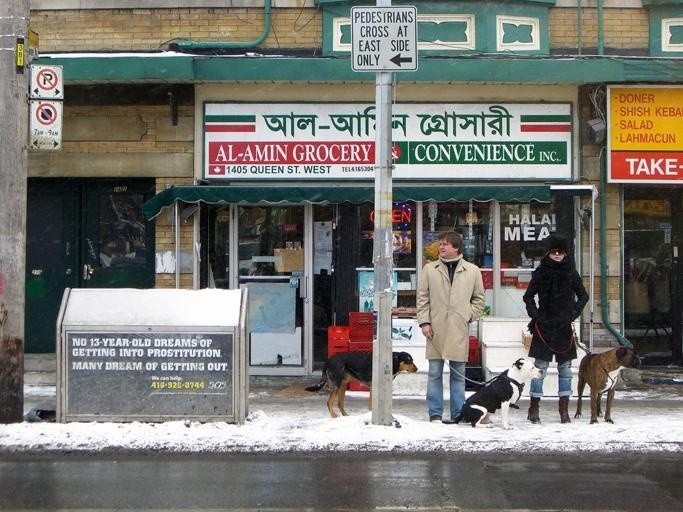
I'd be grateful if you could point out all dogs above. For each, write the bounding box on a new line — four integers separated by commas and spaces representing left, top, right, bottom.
573, 345, 641, 425
442, 357, 544, 430
303, 350, 418, 418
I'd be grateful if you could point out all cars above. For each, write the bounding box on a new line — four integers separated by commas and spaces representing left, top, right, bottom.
238, 240, 261, 278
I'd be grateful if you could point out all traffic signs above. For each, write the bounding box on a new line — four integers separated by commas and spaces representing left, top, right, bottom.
350, 6, 418, 75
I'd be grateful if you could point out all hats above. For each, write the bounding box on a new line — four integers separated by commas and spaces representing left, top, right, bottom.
544, 234, 568, 257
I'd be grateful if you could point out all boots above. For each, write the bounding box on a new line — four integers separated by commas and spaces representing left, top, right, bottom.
558, 395, 571, 423
527, 395, 542, 424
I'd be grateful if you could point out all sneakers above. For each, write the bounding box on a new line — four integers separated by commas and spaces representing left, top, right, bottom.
451, 416, 469, 423
430, 414, 442, 423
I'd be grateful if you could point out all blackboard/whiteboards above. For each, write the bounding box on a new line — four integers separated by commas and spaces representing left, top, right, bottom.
239, 283, 296, 334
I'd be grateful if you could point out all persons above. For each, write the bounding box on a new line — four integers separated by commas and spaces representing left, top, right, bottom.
523, 234, 589, 424
415, 232, 486, 423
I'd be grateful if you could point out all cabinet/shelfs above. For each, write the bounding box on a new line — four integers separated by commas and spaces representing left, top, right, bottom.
478, 313, 597, 396
377, 317, 449, 399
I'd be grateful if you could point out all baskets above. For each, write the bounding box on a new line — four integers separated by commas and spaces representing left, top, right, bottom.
481, 264, 495, 290
501, 270, 518, 285
326, 309, 374, 392
516, 281, 530, 289
466, 335, 481, 364
